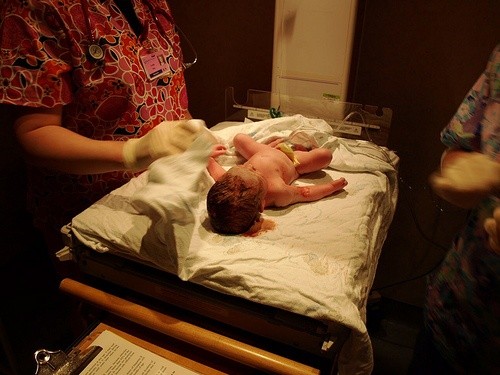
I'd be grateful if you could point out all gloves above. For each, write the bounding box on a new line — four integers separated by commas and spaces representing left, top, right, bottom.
123, 118, 206, 174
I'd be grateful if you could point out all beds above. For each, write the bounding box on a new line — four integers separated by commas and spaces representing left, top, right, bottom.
58, 118, 399, 374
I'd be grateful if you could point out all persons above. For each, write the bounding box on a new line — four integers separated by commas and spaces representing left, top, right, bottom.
0, 0, 206, 337
206, 133, 349, 235
415, 43, 500, 375
